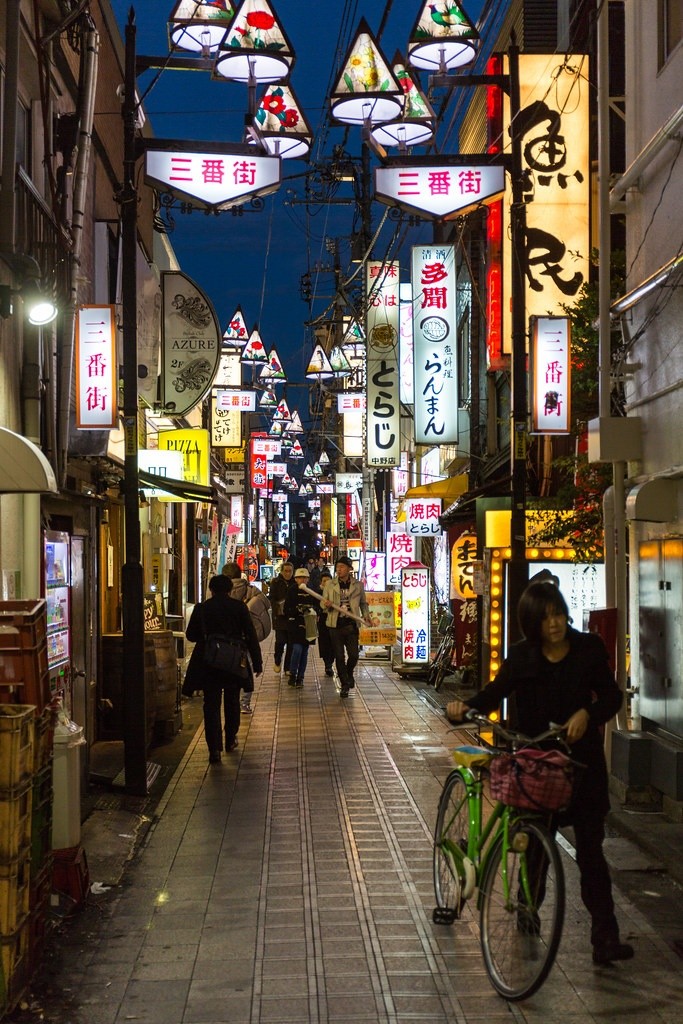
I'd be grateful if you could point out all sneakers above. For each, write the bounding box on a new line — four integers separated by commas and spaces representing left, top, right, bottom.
288, 678, 295, 688
297, 679, 303, 687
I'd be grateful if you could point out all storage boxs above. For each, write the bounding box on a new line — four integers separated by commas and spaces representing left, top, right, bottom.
0, 598, 90, 1005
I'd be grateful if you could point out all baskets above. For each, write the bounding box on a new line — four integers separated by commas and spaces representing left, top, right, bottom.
490, 749, 586, 815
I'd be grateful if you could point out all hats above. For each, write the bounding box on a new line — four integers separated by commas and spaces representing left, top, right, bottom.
294, 568, 309, 577
336, 556, 353, 568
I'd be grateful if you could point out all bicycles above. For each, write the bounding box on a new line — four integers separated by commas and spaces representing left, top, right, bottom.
432, 691, 586, 1007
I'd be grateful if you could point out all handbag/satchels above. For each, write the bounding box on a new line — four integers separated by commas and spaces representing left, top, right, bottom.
489, 749, 585, 812
304, 614, 319, 641
204, 641, 249, 679
242, 586, 271, 642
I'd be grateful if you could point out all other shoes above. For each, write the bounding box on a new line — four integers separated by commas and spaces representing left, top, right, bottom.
593, 943, 634, 965
240, 700, 252, 714
274, 663, 281, 673
325, 667, 334, 676
225, 736, 239, 751
514, 934, 539, 961
284, 671, 291, 676
209, 752, 220, 763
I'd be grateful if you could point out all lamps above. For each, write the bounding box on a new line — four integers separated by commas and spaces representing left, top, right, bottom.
0, 280, 57, 326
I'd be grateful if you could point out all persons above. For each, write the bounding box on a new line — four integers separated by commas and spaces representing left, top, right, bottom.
320, 557, 372, 697
184, 575, 262, 764
445, 582, 635, 963
221, 564, 272, 714
268, 556, 334, 689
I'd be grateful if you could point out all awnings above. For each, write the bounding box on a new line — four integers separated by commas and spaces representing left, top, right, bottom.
101, 452, 216, 495
0, 427, 60, 495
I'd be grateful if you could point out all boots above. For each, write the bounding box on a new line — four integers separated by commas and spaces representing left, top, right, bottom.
347, 659, 357, 689
338, 670, 349, 697
